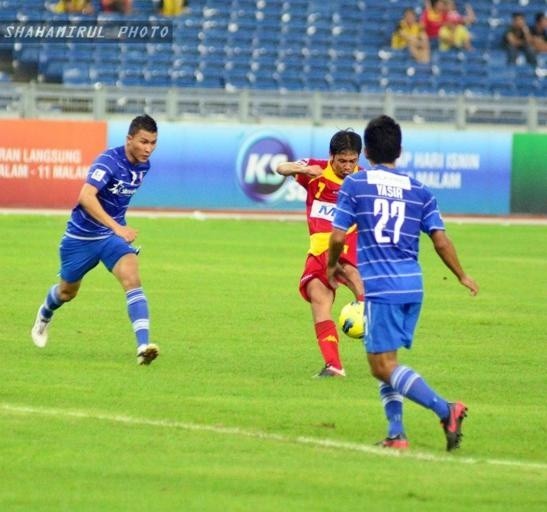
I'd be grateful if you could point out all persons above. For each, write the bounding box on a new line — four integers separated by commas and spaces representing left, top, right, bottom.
54, 0, 188, 16
530, 12, 547, 53
501, 12, 537, 66
392, 0, 476, 64
326, 114, 478, 452
277, 126, 365, 378
32, 114, 159, 366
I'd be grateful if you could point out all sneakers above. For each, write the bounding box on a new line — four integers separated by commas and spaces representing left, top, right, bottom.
314, 363, 346, 378
137, 344, 160, 367
439, 402, 467, 452
375, 431, 411, 449
32, 307, 52, 349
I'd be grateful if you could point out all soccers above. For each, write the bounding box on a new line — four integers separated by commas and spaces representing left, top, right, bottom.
339, 300, 365, 339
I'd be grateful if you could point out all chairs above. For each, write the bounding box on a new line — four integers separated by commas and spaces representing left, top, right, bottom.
0, 0, 547, 95
